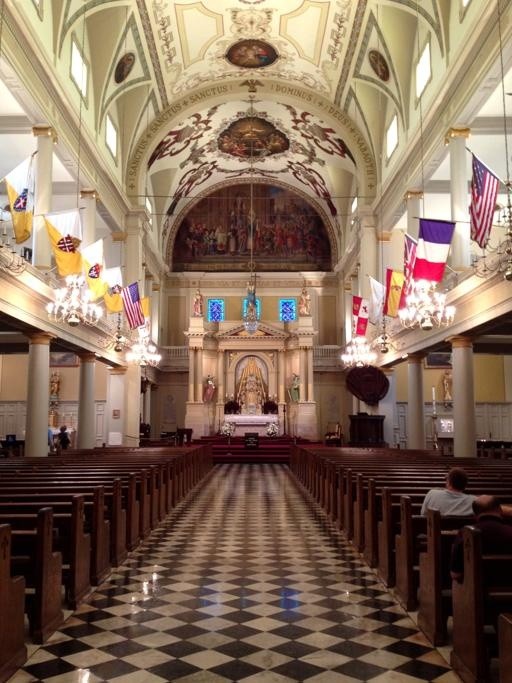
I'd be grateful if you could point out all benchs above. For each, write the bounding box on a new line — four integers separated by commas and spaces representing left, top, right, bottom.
286, 442, 512, 540
1, 444, 215, 540
1, 504, 66, 682
396, 490, 511, 680
352, 468, 512, 587
1, 470, 142, 602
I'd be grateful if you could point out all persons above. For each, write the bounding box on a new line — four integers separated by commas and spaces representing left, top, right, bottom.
57, 425, 71, 450
48, 428, 55, 452
297, 288, 312, 317
448, 494, 512, 581
192, 288, 204, 317
245, 280, 257, 322
202, 356, 301, 415
183, 195, 324, 257
420, 467, 512, 535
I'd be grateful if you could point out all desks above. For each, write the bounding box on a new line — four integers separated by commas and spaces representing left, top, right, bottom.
177, 428, 192, 444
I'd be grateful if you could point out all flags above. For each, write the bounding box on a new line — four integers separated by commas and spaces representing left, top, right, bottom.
5, 155, 37, 245
469, 153, 500, 249
41, 208, 84, 278
81, 238, 109, 302
382, 217, 456, 319
102, 266, 124, 314
367, 276, 386, 326
121, 281, 146, 330
352, 296, 369, 336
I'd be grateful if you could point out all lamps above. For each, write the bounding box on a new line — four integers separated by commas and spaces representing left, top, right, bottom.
125, 199, 160, 367
368, 201, 406, 356
468, 37, 512, 290
47, 141, 104, 331
96, 193, 139, 356
341, 255, 377, 369
395, 101, 458, 334
